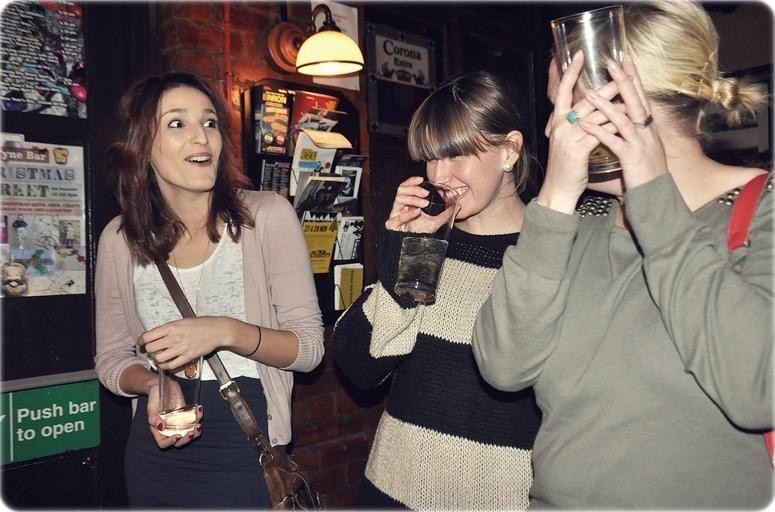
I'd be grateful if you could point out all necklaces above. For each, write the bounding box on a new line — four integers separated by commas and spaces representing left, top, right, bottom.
169, 223, 211, 378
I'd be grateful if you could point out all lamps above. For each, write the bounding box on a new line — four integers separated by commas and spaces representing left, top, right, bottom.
267, 3, 367, 79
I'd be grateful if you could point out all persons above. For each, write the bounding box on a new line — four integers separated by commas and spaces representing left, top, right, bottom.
330, 72, 542, 512
66, 223, 73, 248
13, 214, 28, 250
94, 72, 324, 512
472, 0, 774, 512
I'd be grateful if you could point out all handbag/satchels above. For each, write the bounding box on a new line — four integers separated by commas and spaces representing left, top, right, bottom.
258, 437, 325, 510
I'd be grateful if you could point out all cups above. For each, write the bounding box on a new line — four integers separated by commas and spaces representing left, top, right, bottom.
541, 4, 629, 174
158, 356, 203, 438
394, 181, 458, 304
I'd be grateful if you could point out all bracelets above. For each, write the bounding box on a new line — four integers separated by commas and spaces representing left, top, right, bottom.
244, 325, 261, 357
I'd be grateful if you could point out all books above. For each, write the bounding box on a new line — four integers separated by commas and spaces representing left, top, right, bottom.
261, 106, 367, 310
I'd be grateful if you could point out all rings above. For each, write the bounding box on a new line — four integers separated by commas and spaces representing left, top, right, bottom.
567, 111, 581, 124
633, 115, 653, 127
